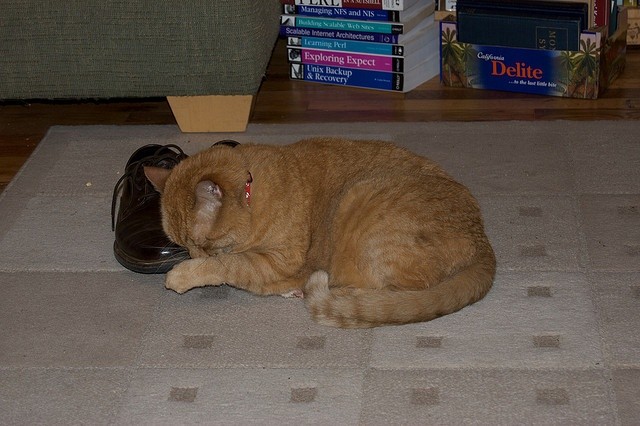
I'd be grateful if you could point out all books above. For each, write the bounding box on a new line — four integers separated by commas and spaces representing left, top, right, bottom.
280, 1, 442, 93
439, 0, 618, 99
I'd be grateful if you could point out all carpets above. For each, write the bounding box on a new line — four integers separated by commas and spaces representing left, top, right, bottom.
1, 120, 639, 426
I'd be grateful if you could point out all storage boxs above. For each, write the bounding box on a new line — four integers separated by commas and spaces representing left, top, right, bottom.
439, 18, 630, 100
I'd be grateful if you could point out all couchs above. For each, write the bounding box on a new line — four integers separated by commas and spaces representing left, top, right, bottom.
1, 0, 287, 134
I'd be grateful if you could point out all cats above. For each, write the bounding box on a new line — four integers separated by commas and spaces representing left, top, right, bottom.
143, 136, 496, 327
